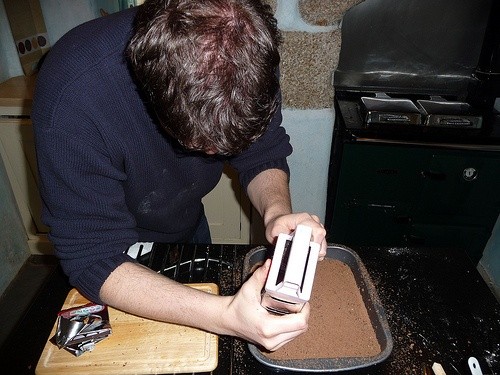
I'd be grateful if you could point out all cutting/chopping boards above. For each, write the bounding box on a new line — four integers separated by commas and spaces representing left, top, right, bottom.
35, 283, 219, 375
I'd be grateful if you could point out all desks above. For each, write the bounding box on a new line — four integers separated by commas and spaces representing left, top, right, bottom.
0, 242, 500, 375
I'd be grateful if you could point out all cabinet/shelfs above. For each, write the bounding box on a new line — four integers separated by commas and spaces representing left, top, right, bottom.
324, 94, 500, 260
0, 75, 54, 255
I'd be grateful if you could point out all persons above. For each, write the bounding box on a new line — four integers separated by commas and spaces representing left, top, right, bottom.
32, 0, 328, 352
